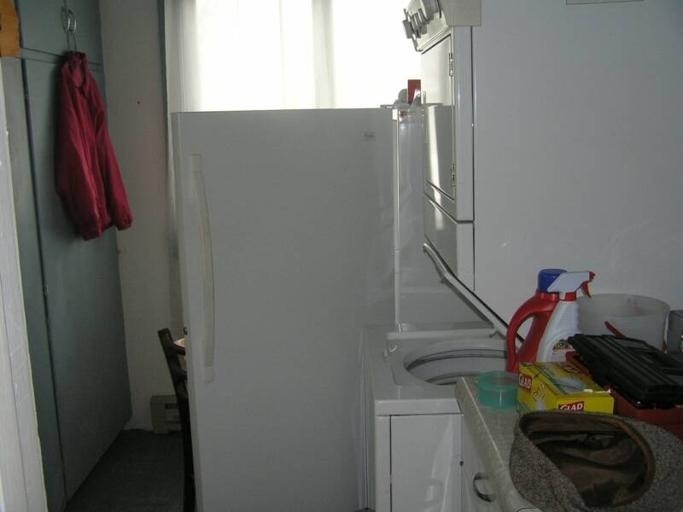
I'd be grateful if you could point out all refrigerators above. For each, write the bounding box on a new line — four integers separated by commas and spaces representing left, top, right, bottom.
169, 106, 436, 512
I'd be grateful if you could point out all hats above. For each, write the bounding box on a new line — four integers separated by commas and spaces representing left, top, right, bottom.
510, 408, 683, 512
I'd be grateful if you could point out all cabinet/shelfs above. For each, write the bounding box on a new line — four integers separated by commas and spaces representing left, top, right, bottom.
21, 57, 133, 502
459, 414, 503, 512
15, 0, 103, 66
1, 56, 68, 512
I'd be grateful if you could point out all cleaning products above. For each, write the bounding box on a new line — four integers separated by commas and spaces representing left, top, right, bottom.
538, 270, 595, 365
505, 268, 567, 371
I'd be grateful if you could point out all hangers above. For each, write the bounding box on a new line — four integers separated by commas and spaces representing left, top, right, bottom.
66, 28, 82, 65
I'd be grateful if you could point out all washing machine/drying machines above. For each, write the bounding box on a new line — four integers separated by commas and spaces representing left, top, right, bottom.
367, 243, 523, 510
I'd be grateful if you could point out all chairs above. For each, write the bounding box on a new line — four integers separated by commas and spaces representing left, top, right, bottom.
157, 326, 197, 512
